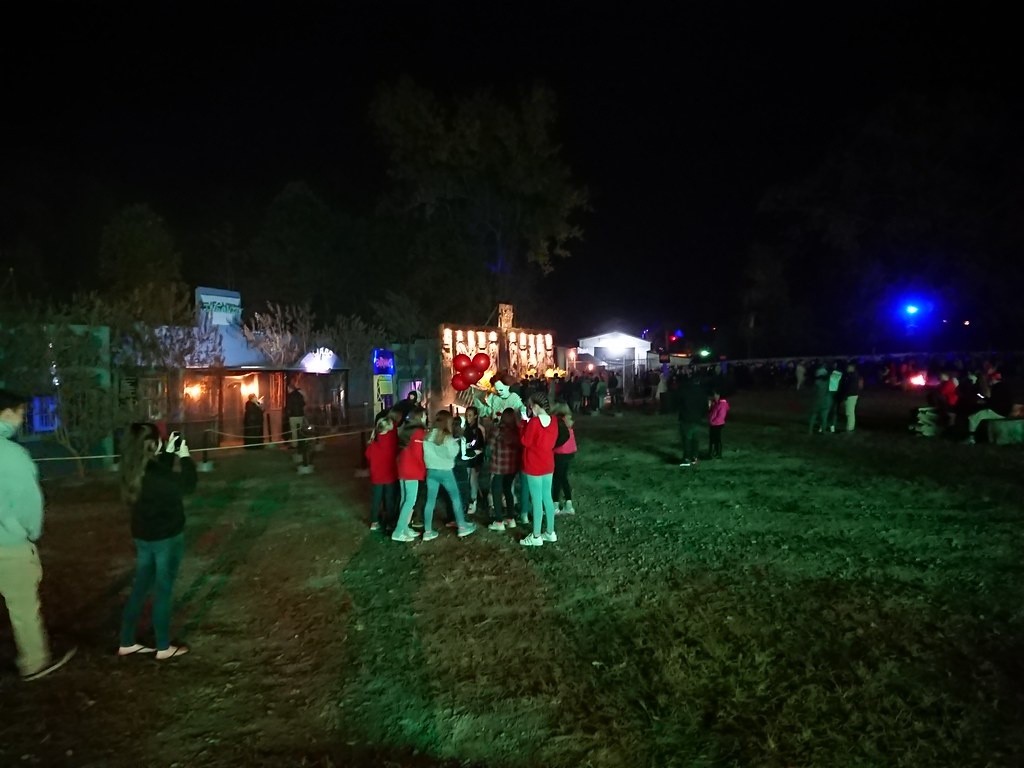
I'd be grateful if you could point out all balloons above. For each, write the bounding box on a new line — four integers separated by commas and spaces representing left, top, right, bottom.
451, 353, 490, 390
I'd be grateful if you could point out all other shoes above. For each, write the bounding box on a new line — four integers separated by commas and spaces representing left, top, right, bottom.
488, 521, 506, 530
466, 495, 574, 514
679, 459, 697, 466
505, 518, 516, 528
370, 522, 380, 530
22, 650, 76, 682
386, 522, 396, 530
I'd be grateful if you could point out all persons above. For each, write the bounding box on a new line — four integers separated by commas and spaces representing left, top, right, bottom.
363, 391, 578, 546
679, 374, 730, 467
939, 360, 1013, 444
117, 422, 199, 660
795, 359, 806, 390
471, 372, 526, 427
0, 388, 75, 682
285, 385, 306, 449
648, 367, 676, 400
807, 361, 859, 435
520, 370, 623, 413
243, 393, 264, 450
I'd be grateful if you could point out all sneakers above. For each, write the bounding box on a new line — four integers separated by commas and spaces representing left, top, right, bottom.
459, 523, 476, 537
542, 531, 557, 542
392, 533, 414, 541
520, 533, 543, 546
406, 527, 421, 537
423, 531, 439, 541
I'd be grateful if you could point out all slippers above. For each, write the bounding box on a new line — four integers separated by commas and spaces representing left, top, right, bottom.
155, 644, 191, 660
117, 645, 158, 656
